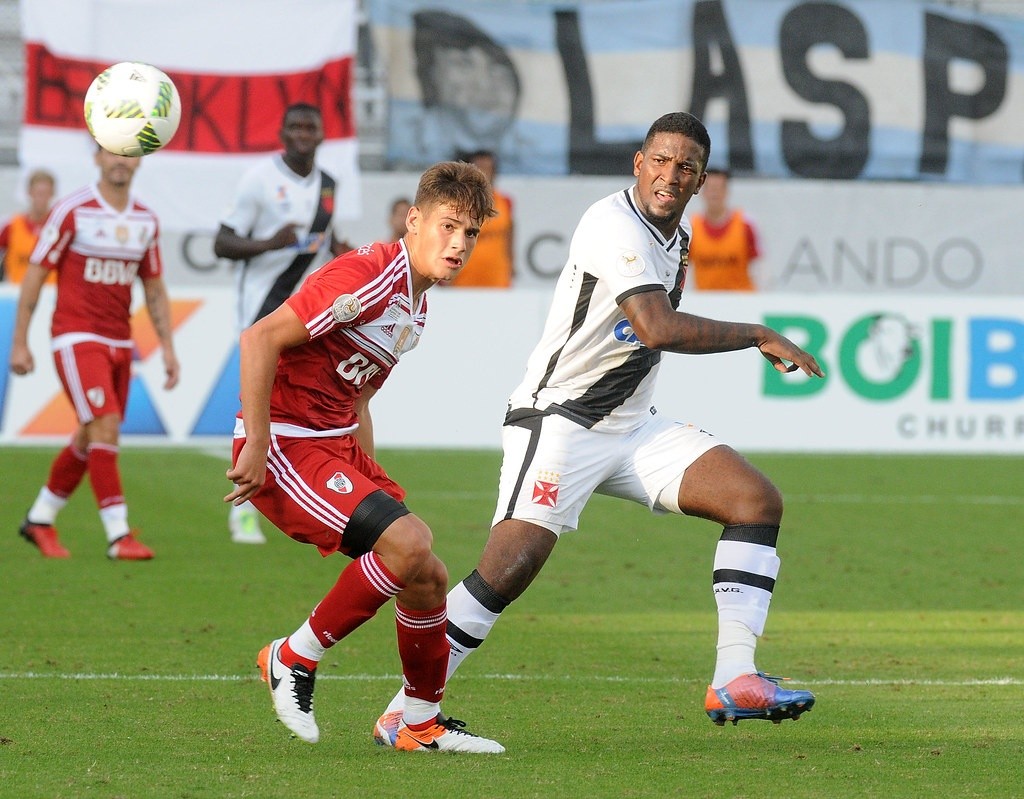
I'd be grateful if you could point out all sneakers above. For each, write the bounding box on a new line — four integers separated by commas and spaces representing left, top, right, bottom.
228, 509, 270, 546
108, 533, 155, 561
256, 636, 321, 745
19, 519, 72, 561
372, 708, 404, 746
395, 717, 507, 754
705, 671, 815, 725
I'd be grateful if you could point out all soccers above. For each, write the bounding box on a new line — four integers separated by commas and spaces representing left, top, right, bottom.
84, 59, 182, 159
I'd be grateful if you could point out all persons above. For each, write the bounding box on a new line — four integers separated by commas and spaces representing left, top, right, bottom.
687, 167, 758, 290
9, 144, 181, 562
372, 111, 826, 747
440, 151, 519, 286
387, 198, 414, 243
223, 161, 504, 753
214, 102, 358, 543
0, 174, 58, 284
